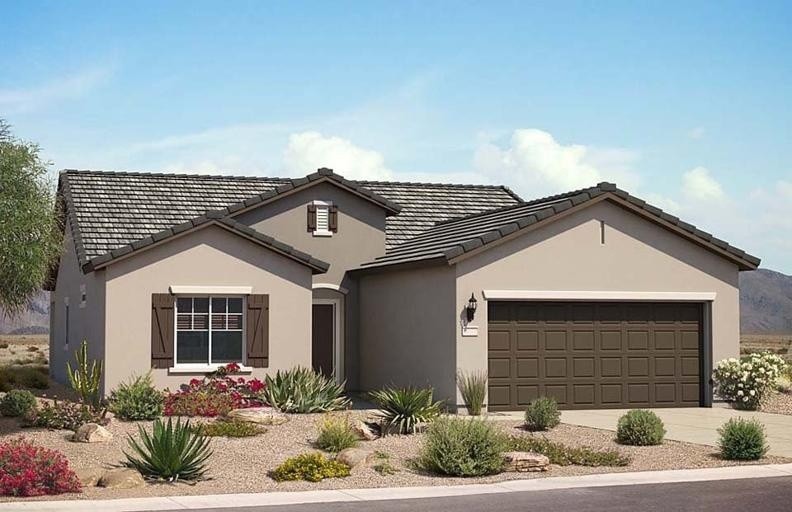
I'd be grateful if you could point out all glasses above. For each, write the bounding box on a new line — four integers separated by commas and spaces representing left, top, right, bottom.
467, 292, 477, 322
